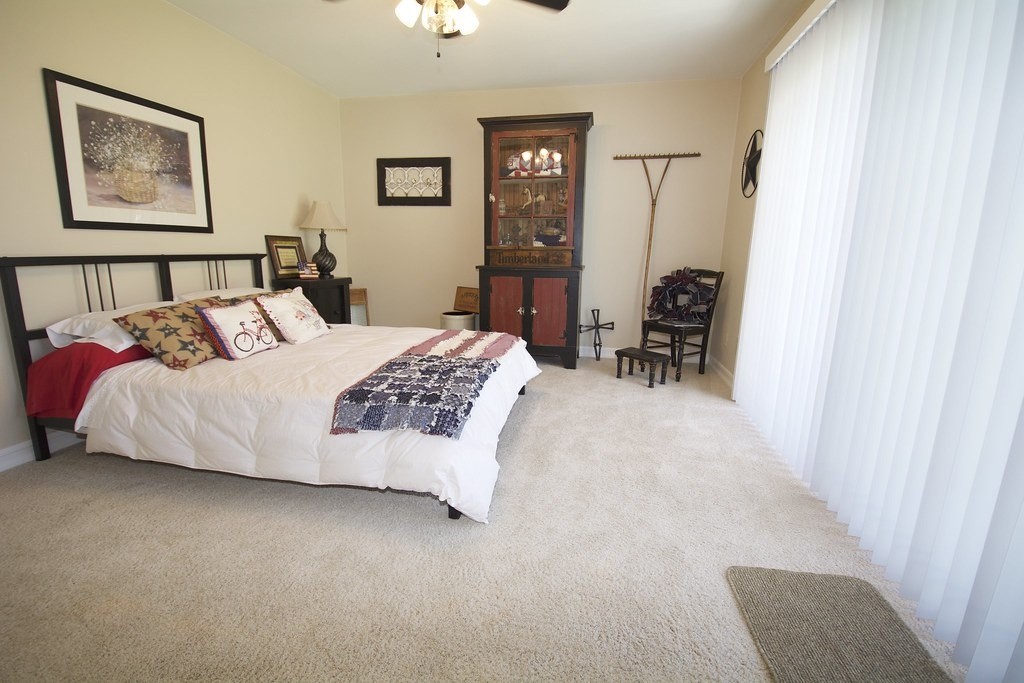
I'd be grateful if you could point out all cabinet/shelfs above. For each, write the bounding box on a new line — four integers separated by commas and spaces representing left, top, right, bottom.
477, 112, 594, 369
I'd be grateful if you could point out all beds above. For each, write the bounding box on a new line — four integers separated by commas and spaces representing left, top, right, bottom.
0, 253, 542, 524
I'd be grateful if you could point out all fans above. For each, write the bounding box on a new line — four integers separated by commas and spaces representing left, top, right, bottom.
321, 0, 569, 39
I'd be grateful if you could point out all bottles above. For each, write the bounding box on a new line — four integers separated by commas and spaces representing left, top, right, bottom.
557, 187, 568, 214
499, 198, 507, 216
504, 232, 512, 247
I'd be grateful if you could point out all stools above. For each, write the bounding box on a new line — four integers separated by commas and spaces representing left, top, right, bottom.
615, 347, 672, 389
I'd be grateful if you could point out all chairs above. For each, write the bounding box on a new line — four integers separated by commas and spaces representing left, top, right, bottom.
639, 269, 724, 382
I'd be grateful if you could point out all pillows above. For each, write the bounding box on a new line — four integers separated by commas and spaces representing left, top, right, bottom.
46, 301, 178, 352
197, 299, 279, 361
257, 286, 330, 346
113, 295, 228, 370
178, 288, 273, 303
228, 288, 332, 342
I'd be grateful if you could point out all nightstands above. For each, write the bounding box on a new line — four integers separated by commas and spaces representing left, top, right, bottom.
272, 277, 351, 324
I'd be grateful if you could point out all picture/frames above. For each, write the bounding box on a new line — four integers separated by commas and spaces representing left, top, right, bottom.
42, 67, 214, 234
265, 235, 307, 279
376, 158, 452, 206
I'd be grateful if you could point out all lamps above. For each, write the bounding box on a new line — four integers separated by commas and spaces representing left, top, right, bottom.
395, 0, 491, 57
299, 200, 348, 279
